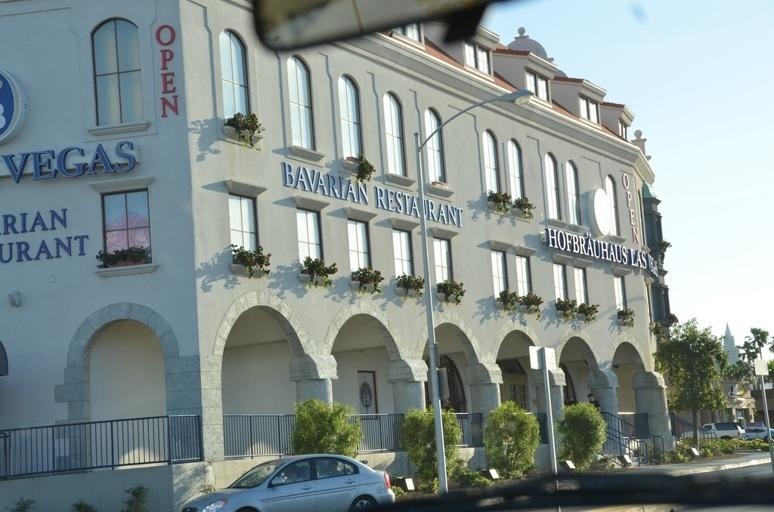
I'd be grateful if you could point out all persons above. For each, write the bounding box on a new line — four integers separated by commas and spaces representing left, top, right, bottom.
270, 461, 342, 481
587, 393, 602, 413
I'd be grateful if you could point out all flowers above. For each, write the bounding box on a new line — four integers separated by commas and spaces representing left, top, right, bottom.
488, 189, 512, 216
355, 154, 376, 184
230, 242, 271, 281
433, 279, 467, 307
96, 245, 149, 269
393, 272, 427, 302
302, 256, 339, 291
224, 112, 266, 151
350, 267, 384, 296
513, 195, 536, 222
495, 289, 636, 328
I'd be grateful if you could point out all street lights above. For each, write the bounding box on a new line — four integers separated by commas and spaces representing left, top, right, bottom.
412, 84, 538, 500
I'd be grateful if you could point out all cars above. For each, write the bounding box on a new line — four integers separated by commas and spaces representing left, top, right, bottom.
681, 421, 774, 444
178, 451, 400, 510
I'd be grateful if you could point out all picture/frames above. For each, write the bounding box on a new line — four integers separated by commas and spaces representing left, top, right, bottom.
358, 370, 378, 420
